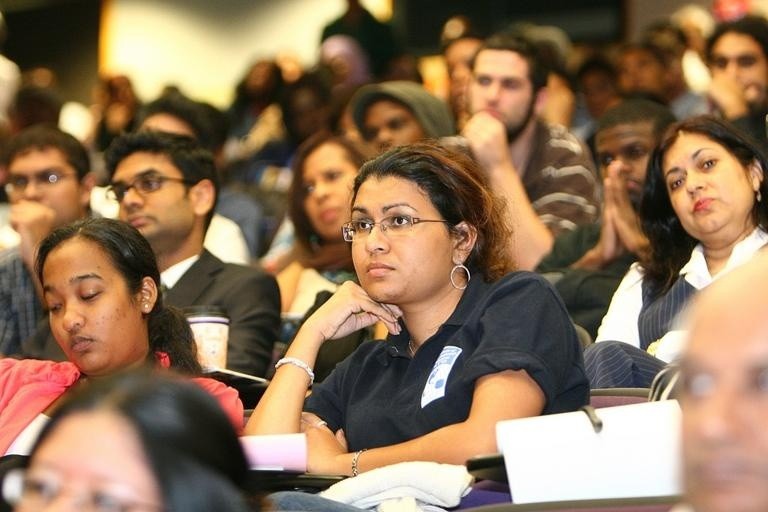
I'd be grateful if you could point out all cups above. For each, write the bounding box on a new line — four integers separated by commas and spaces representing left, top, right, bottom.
180, 305, 231, 372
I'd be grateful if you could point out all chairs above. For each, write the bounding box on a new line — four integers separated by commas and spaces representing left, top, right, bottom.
589, 389, 648, 409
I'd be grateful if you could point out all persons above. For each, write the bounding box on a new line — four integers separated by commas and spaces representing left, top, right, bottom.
582, 113, 766, 408
243, 139, 589, 511
0, 217, 246, 512
11, 368, 276, 511
0, 0, 768, 380
675, 243, 767, 512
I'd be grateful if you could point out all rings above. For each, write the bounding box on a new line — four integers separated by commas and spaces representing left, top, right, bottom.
315, 420, 328, 427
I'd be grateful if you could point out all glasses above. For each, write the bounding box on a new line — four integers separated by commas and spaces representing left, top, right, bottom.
342, 215, 447, 242
105, 174, 188, 201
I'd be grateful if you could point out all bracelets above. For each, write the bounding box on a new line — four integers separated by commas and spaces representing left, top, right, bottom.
351, 448, 367, 477
